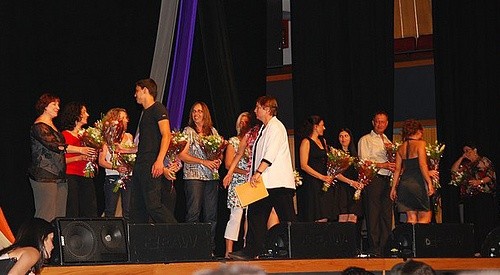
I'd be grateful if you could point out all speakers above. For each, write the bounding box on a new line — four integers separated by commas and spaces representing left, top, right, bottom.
46, 217, 213, 266
262, 221, 358, 260
480, 225, 500, 258
384, 222, 475, 258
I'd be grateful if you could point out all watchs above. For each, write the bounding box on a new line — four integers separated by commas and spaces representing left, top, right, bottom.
480, 179, 484, 185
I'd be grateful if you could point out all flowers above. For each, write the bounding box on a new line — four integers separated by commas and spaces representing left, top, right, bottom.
426, 139, 446, 189
386, 142, 402, 185
198, 133, 228, 177
352, 158, 378, 198
165, 130, 190, 186
448, 169, 490, 190
84, 118, 137, 191
322, 146, 354, 191
294, 170, 303, 187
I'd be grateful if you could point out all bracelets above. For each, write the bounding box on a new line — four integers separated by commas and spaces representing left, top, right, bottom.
350, 180, 354, 186
256, 170, 262, 174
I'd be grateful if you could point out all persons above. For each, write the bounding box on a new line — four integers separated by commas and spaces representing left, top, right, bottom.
108, 78, 178, 225
0, 217, 55, 275
228, 95, 297, 261
161, 156, 182, 215
451, 141, 496, 256
299, 116, 338, 222
24, 94, 96, 223
357, 110, 440, 257
98, 108, 134, 228
177, 101, 224, 260
223, 111, 252, 260
332, 127, 361, 225
60, 102, 98, 219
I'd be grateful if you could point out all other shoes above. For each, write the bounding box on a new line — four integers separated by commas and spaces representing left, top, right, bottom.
260, 251, 289, 259
242, 256, 259, 261
225, 253, 245, 260
